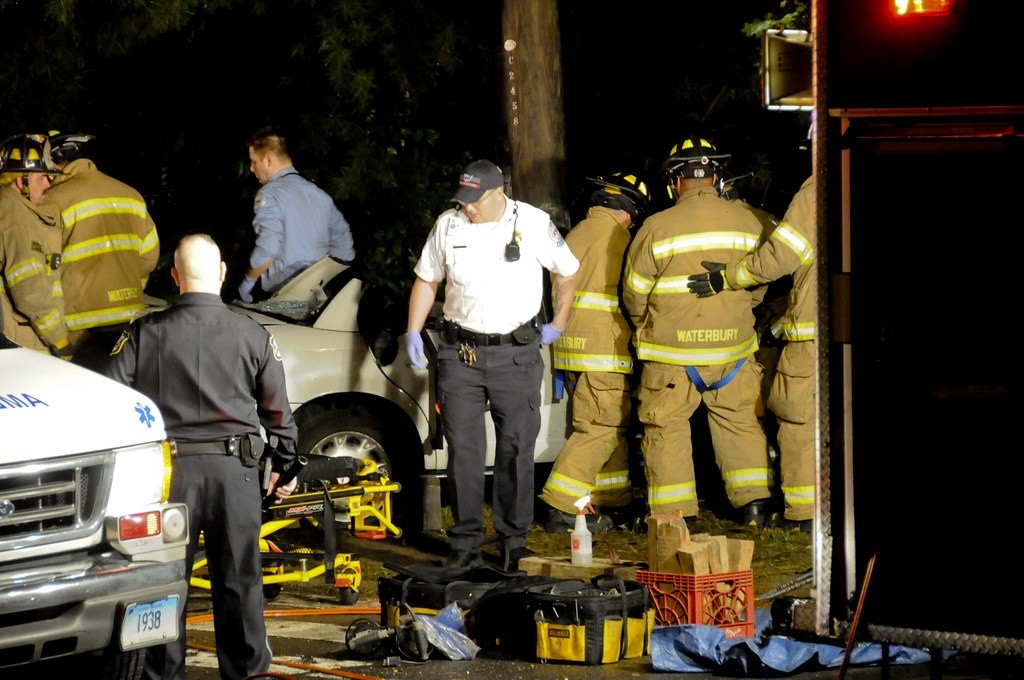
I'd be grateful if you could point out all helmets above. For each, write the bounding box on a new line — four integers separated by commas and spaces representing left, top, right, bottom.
0, 134, 64, 174
663, 139, 720, 169
596, 172, 651, 211
47, 130, 93, 151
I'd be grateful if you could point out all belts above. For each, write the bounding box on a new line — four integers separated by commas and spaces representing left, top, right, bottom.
176, 440, 242, 457
460, 321, 532, 345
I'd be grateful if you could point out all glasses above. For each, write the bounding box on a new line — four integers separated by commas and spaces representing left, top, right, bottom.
458, 189, 495, 205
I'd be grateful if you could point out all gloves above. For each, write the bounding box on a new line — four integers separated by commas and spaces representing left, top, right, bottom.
405, 332, 428, 370
687, 261, 733, 299
239, 274, 256, 303
540, 323, 562, 346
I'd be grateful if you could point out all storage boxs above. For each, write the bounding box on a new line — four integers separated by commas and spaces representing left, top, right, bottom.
636, 569, 755, 639
518, 555, 648, 581
524, 577, 648, 666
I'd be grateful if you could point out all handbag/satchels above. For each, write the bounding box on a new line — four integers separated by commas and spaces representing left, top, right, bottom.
378, 549, 655, 665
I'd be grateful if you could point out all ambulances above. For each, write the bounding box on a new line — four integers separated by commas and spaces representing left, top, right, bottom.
1, 332, 193, 680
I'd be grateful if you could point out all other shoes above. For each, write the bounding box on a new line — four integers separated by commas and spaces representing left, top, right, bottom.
771, 512, 813, 534
634, 512, 696, 528
746, 502, 765, 522
549, 509, 611, 531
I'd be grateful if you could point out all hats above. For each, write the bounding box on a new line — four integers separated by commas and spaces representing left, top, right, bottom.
450, 160, 504, 202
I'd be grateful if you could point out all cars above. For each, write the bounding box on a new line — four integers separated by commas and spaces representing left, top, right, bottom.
136, 253, 573, 539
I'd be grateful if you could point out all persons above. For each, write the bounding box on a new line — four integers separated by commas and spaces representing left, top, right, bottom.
406, 160, 580, 577
238, 132, 356, 302
104, 235, 298, 680
0, 125, 160, 373
687, 173, 816, 537
620, 133, 804, 535
537, 172, 654, 531
